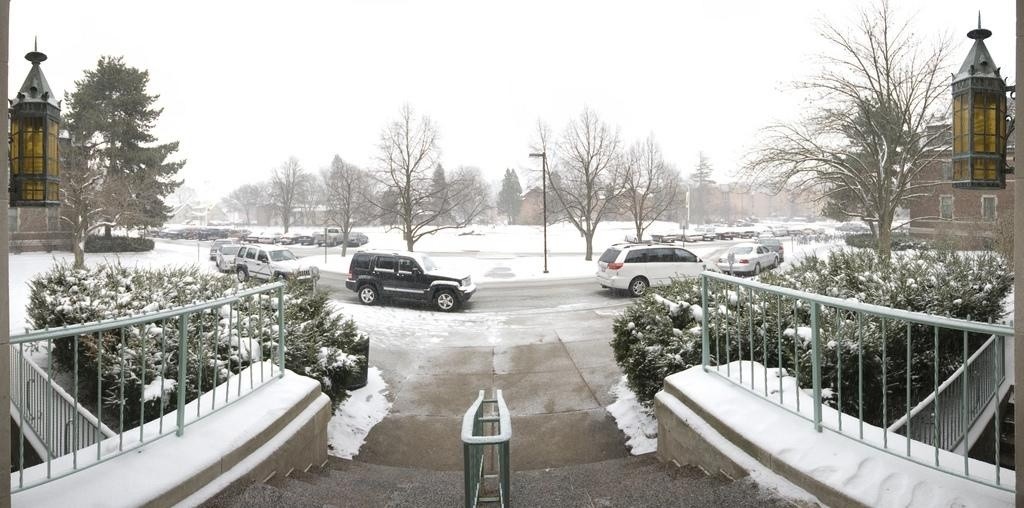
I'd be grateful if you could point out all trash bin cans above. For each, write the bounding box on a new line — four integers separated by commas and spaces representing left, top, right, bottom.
344, 330, 369, 390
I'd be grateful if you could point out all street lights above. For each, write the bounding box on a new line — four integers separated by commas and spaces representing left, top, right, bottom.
528, 151, 550, 273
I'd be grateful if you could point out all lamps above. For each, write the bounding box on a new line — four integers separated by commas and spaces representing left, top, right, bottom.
11, 33, 66, 210
950, 9, 1016, 190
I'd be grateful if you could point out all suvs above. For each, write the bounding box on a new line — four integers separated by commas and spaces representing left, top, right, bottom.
594, 240, 723, 297
345, 248, 477, 313
232, 241, 319, 289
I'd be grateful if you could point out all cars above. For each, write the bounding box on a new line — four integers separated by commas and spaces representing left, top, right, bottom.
624, 213, 870, 243
718, 243, 781, 275
136, 225, 369, 247
208, 236, 243, 272
757, 238, 784, 263
